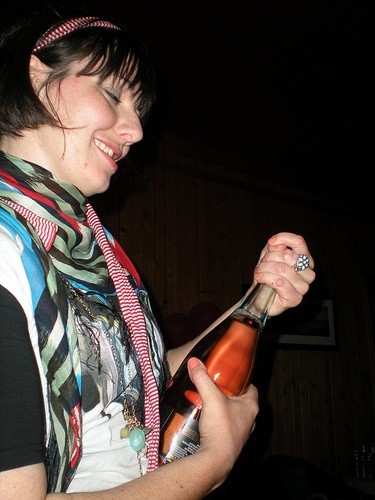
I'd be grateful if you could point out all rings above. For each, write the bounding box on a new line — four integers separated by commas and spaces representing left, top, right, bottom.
292, 253, 309, 273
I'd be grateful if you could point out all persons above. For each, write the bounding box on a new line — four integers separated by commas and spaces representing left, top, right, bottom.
0, 0, 316, 500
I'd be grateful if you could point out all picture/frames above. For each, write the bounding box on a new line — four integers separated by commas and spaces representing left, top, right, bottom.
274, 296, 339, 349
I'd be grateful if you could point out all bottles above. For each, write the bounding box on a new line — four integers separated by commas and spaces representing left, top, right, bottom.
160, 282, 278, 468
